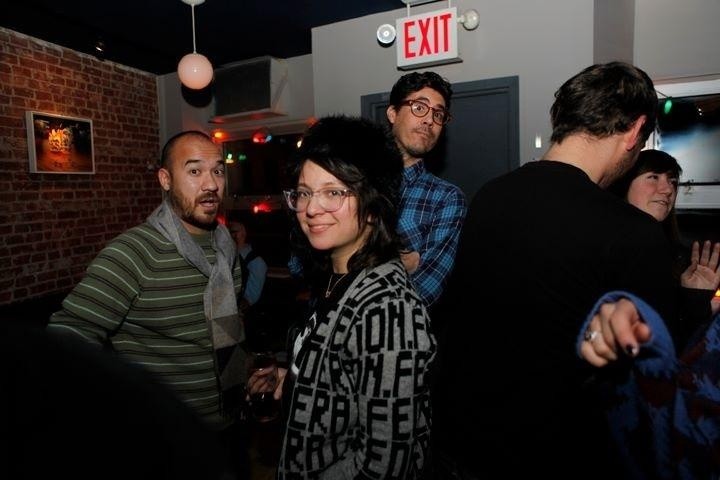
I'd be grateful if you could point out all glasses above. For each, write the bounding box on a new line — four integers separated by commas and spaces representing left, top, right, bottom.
284, 188, 355, 213
402, 98, 453, 127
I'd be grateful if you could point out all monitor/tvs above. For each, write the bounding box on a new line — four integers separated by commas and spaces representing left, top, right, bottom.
222, 132, 316, 214
641, 77, 720, 209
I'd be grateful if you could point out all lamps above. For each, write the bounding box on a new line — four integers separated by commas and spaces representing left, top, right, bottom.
462, 9, 480, 30
376, 24, 396, 45
177, 0, 214, 90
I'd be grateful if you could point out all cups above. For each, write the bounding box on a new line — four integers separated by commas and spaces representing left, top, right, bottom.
246, 365, 282, 423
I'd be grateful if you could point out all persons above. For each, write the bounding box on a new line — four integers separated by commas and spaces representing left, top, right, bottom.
245, 111, 437, 478
44, 130, 241, 426
227, 221, 267, 312
288, 70, 470, 305
459, 62, 720, 479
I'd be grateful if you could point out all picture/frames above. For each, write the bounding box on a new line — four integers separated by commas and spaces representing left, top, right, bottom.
25, 110, 96, 175
